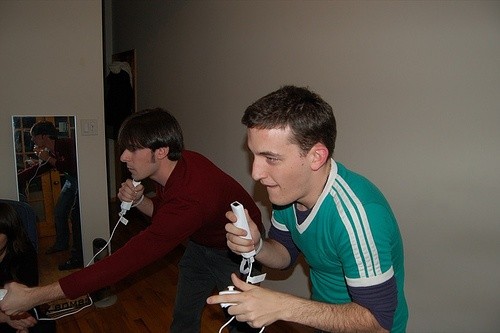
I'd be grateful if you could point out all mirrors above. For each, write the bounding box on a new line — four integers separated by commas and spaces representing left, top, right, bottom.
11, 115, 88, 315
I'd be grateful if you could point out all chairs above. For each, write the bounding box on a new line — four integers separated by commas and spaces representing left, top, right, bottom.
0, 199, 39, 288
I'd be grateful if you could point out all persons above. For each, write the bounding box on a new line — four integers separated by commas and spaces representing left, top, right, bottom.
0, 107, 264, 333
0, 201, 37, 333
205, 87, 409, 333
17, 120, 83, 271
